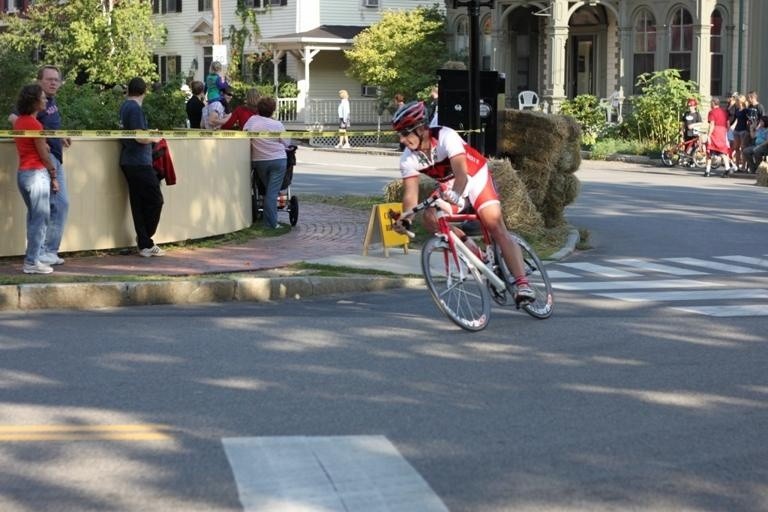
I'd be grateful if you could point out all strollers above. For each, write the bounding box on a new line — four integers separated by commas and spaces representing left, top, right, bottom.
250, 144, 298, 228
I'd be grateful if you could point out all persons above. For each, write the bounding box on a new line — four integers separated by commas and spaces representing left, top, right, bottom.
680, 90, 768, 177
392, 99, 536, 310
14, 84, 59, 273
120, 77, 165, 257
9, 65, 71, 264
418, 98, 429, 123
395, 93, 405, 151
186, 61, 294, 228
428, 86, 438, 122
334, 89, 351, 147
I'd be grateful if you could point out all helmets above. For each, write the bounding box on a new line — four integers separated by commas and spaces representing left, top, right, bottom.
687, 99, 698, 107
391, 101, 430, 132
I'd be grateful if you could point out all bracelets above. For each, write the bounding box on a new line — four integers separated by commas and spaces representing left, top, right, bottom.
50, 176, 57, 178
49, 167, 57, 171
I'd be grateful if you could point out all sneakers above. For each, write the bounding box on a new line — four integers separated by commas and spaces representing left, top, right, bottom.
722, 167, 734, 178
683, 161, 697, 168
263, 222, 285, 231
513, 284, 537, 307
735, 163, 758, 174
334, 142, 350, 148
23, 255, 64, 273
704, 172, 710, 177
135, 235, 166, 257
465, 260, 495, 281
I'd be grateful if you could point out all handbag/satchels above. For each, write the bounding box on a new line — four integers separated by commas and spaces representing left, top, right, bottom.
338, 119, 351, 128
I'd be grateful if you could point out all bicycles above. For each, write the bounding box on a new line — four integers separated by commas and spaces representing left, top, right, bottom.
655, 126, 714, 174
387, 178, 556, 332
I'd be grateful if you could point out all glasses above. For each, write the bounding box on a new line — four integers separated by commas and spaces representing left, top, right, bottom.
396, 123, 426, 139
223, 91, 235, 97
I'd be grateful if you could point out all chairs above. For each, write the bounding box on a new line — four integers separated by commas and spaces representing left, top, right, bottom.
518, 90, 540, 110
600, 90, 618, 122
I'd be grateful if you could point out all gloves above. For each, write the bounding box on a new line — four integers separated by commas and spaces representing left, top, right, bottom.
392, 219, 411, 235
439, 188, 459, 204
